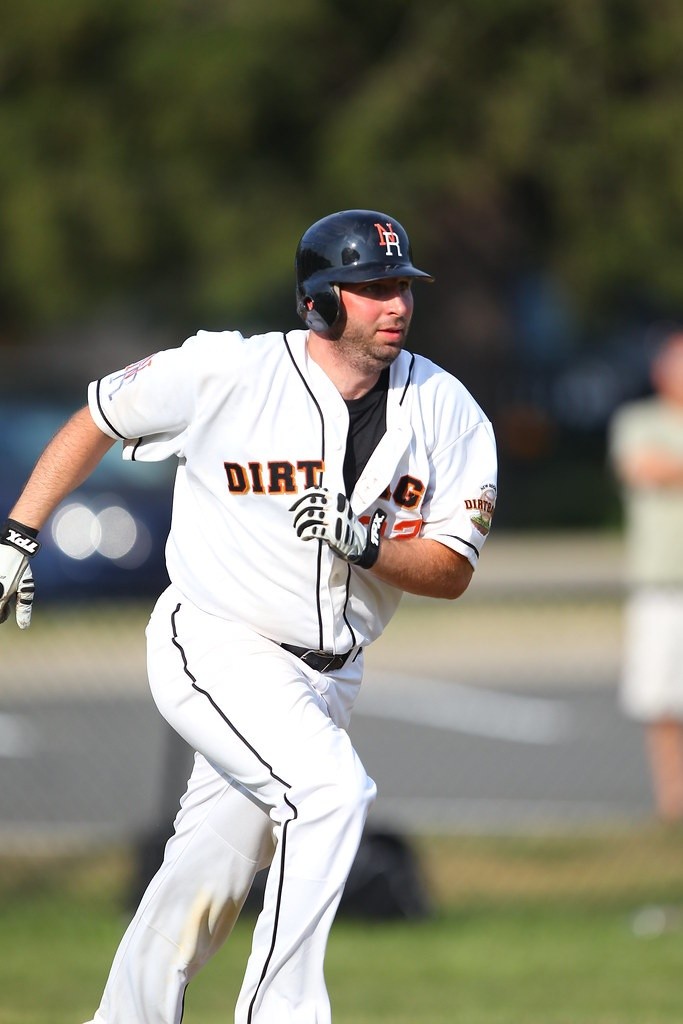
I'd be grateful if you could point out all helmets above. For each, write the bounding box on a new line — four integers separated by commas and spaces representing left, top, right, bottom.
291, 207, 437, 335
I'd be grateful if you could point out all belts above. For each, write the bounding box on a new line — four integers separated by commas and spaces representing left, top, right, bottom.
279, 642, 365, 673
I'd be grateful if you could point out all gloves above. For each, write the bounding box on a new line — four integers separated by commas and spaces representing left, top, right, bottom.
0, 517, 42, 634
287, 484, 388, 573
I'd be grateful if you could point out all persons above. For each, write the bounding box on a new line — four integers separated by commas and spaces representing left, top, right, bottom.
1, 210, 501, 1024
607, 323, 683, 934
